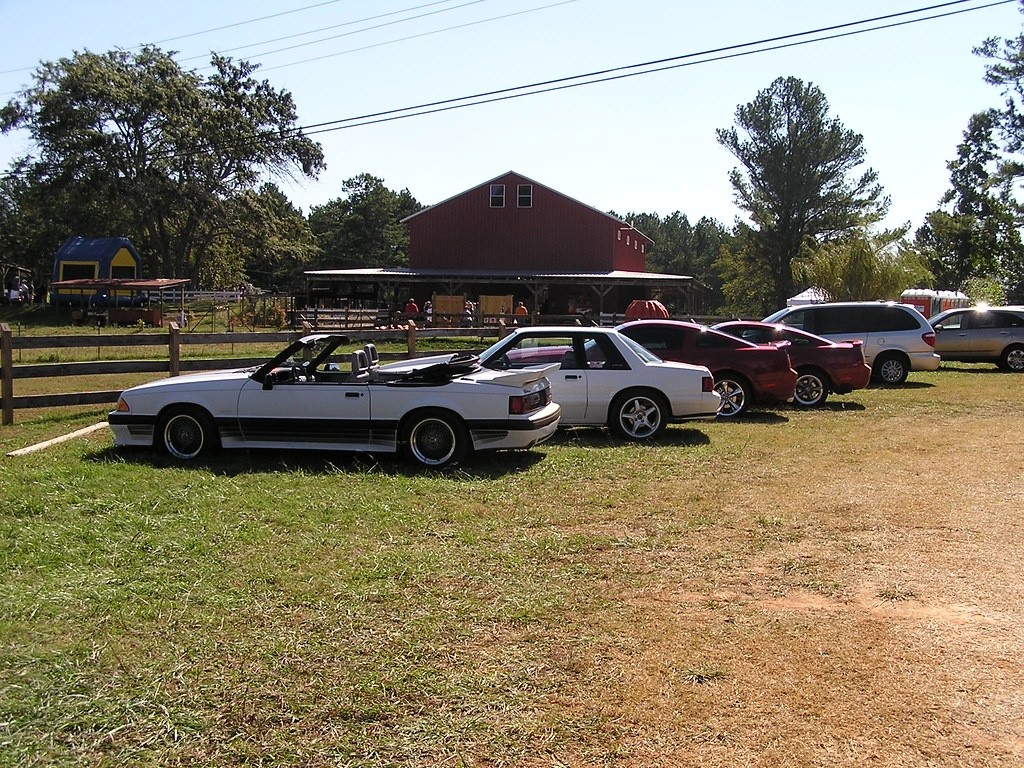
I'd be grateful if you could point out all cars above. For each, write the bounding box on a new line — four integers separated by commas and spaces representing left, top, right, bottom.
378, 327, 722, 442
505, 320, 798, 418
710, 321, 872, 407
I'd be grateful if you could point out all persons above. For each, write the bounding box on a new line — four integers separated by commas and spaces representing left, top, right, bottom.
12, 275, 36, 306
405, 297, 419, 323
515, 302, 528, 326
424, 300, 433, 323
465, 300, 479, 319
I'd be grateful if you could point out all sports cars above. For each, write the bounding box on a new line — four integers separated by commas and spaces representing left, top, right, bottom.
107, 331, 563, 472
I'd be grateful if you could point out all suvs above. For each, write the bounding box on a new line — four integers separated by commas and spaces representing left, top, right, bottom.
926, 306, 1024, 372
744, 299, 941, 385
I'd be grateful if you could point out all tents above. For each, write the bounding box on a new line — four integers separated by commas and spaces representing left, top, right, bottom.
786, 284, 840, 325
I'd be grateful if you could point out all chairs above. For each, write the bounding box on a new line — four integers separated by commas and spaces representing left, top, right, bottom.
345, 350, 370, 384
364, 344, 380, 370
561, 351, 579, 368
